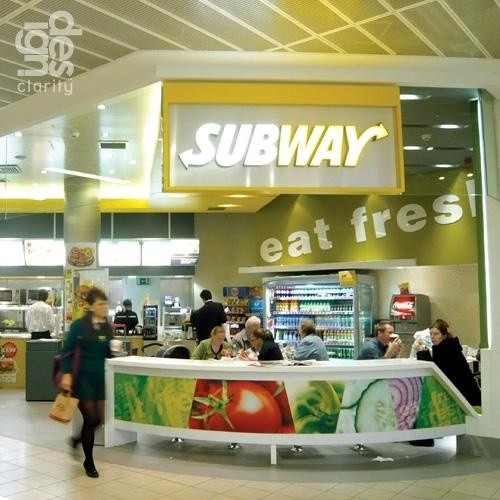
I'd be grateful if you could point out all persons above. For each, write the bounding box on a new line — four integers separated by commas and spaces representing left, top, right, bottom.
286, 320, 329, 361
26, 289, 56, 339
358, 322, 403, 359
410, 319, 449, 358
190, 326, 233, 360
473, 344, 481, 391
114, 300, 138, 335
196, 290, 226, 344
238, 328, 283, 360
409, 323, 481, 447
232, 316, 261, 351
60, 287, 125, 477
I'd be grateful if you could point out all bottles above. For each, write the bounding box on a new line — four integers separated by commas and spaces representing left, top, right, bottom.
146, 309, 157, 337
272, 285, 355, 360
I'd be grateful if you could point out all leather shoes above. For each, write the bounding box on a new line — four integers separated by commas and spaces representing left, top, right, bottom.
83, 460, 99, 478
68, 436, 84, 463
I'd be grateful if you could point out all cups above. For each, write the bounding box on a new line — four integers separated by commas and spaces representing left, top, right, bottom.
110, 339, 123, 353
388, 333, 398, 343
461, 344, 468, 358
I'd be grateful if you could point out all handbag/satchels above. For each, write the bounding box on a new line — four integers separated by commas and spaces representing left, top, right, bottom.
50, 343, 81, 389
48, 394, 80, 425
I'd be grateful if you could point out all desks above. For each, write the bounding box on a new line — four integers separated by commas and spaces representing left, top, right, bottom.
217, 346, 336, 361
103, 354, 477, 465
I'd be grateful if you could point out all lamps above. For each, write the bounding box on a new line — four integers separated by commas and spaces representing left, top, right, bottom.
0, 164, 23, 175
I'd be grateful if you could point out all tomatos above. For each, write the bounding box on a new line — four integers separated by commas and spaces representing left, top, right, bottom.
188, 379, 295, 434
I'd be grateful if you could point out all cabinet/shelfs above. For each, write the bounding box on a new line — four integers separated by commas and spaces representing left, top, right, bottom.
221, 303, 248, 329
0, 289, 64, 333
162, 306, 186, 339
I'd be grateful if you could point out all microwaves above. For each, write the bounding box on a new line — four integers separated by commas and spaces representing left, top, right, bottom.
0, 286, 57, 306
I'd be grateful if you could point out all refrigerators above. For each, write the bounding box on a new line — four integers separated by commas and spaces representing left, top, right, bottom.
144, 304, 158, 340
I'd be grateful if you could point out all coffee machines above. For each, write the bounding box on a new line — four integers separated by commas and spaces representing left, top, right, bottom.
181, 322, 195, 340
164, 307, 184, 327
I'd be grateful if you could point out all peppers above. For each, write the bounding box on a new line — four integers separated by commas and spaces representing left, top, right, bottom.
293, 381, 344, 433
